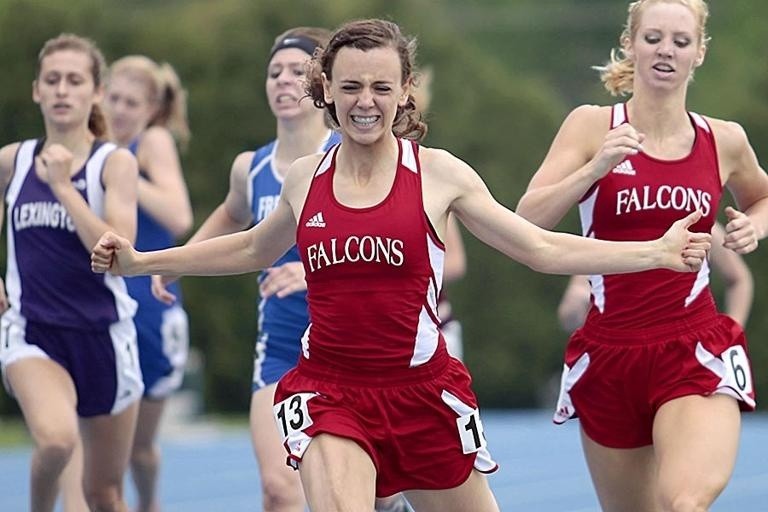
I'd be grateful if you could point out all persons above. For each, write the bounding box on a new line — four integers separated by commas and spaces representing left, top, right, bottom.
513, 1, 767, 511
557, 221, 754, 332
63, 54, 192, 510
152, 25, 410, 508
1, 32, 144, 512
393, 67, 467, 363
90, 19, 712, 510
